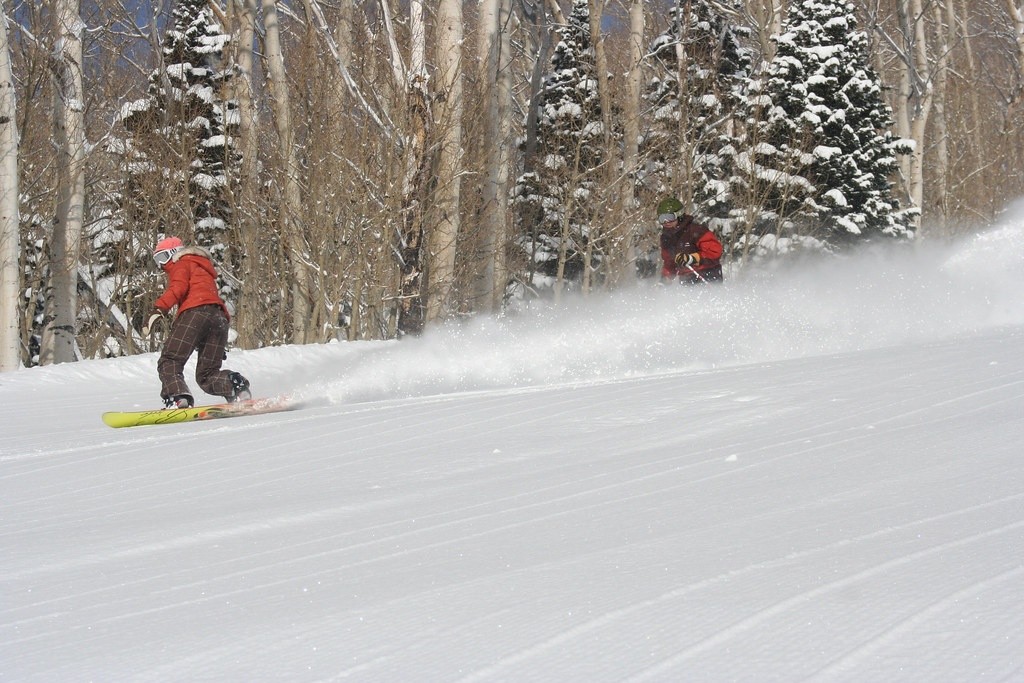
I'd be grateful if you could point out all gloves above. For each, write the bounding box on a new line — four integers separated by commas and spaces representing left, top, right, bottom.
674, 252, 700, 267
150, 308, 167, 320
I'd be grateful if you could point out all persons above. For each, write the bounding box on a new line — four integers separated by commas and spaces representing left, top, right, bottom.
142, 237, 253, 410
657, 198, 724, 286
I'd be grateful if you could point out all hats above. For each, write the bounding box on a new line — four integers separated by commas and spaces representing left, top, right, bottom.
154, 237, 183, 250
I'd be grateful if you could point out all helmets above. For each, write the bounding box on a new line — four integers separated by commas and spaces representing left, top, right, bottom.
657, 198, 683, 213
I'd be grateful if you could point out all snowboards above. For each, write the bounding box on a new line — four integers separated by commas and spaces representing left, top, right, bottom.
99, 392, 297, 428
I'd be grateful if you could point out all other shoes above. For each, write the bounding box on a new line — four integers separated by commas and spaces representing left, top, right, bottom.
225, 373, 252, 402
166, 394, 194, 409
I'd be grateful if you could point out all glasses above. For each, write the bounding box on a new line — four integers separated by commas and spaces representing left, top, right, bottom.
153, 246, 183, 270
658, 209, 683, 224
